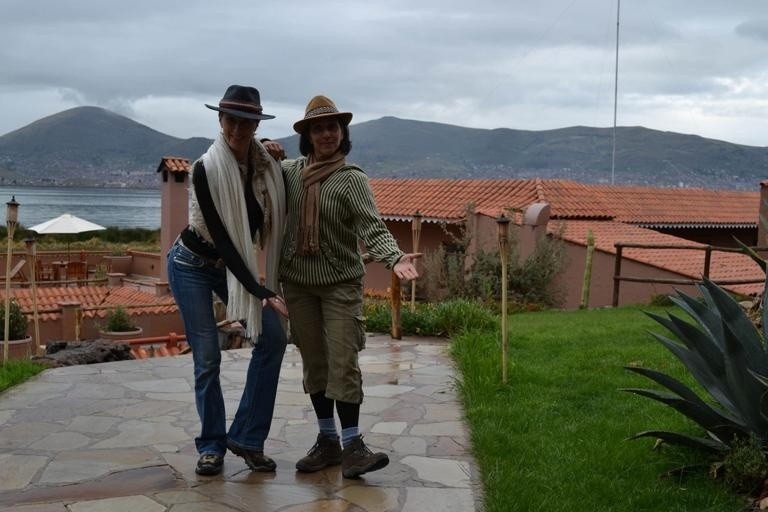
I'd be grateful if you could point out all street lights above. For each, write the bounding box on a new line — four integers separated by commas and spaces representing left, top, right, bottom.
24, 237, 41, 357
4, 195, 21, 366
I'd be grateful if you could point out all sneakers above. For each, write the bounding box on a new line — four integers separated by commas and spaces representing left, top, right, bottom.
195, 451, 224, 475
226, 436, 277, 472
296, 433, 342, 471
342, 438, 389, 479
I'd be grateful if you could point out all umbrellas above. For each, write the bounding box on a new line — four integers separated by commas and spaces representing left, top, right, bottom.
26, 214, 106, 273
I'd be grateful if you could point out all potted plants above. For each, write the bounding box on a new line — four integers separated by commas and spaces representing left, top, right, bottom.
1, 298, 33, 362
93, 303, 145, 341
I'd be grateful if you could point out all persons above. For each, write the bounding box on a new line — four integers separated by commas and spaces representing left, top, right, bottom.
254, 96, 424, 479
167, 85, 289, 476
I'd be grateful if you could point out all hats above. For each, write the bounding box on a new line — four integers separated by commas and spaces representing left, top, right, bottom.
294, 95, 353, 132
205, 85, 276, 120
